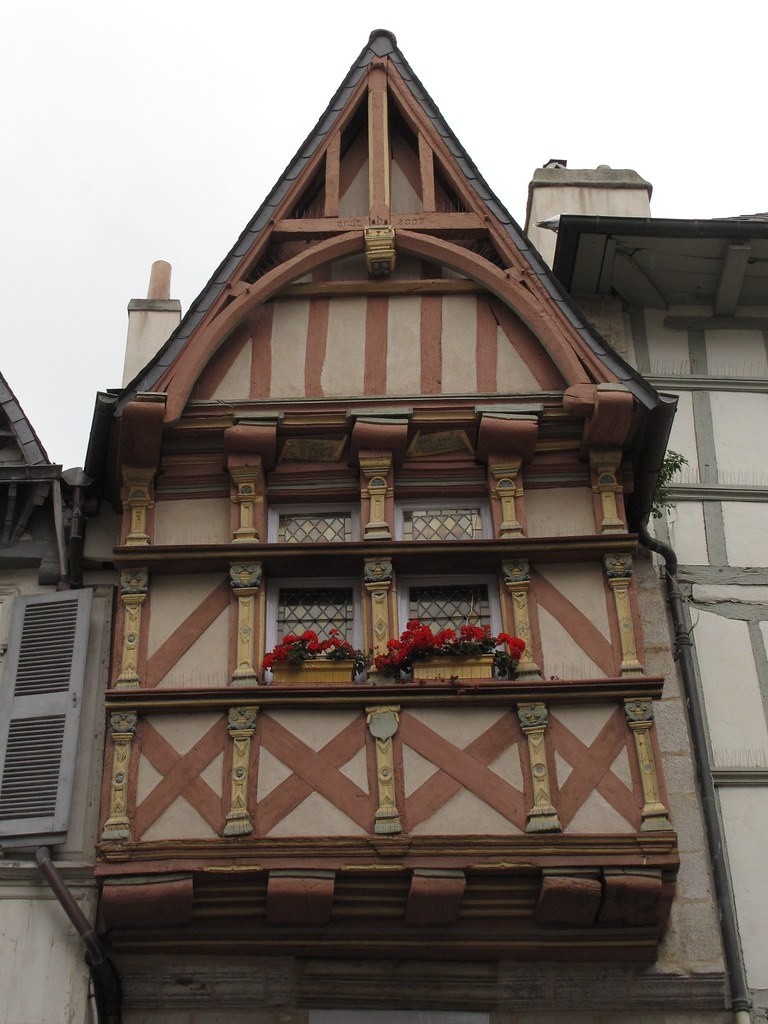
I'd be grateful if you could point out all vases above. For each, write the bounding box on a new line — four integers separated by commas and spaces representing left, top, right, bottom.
412, 654, 496, 679
272, 660, 355, 683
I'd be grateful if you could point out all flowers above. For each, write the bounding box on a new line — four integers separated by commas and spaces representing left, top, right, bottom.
262, 628, 374, 677
374, 619, 527, 677
